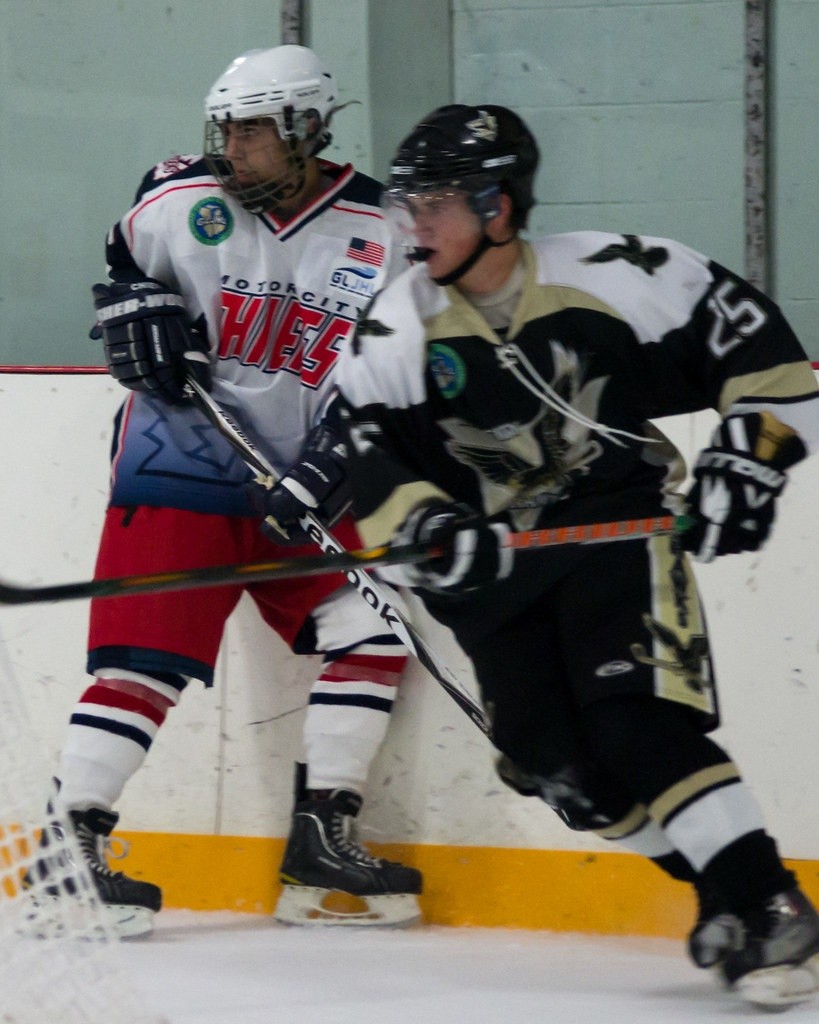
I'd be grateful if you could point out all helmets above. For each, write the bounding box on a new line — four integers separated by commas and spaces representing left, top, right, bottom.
381, 102, 540, 242
200, 40, 340, 207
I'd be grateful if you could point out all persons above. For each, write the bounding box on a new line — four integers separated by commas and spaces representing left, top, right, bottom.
18, 42, 428, 940
336, 104, 818, 1011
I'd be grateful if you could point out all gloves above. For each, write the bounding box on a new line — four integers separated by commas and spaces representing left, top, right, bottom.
378, 494, 515, 602
672, 415, 808, 561
87, 276, 208, 403
262, 419, 385, 547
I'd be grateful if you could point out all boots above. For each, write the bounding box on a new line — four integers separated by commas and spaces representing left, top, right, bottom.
647, 831, 818, 1007
16, 778, 161, 941
276, 761, 426, 929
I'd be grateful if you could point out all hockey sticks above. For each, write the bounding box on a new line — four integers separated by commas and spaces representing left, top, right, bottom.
1, 516, 694, 606
185, 376, 506, 754
